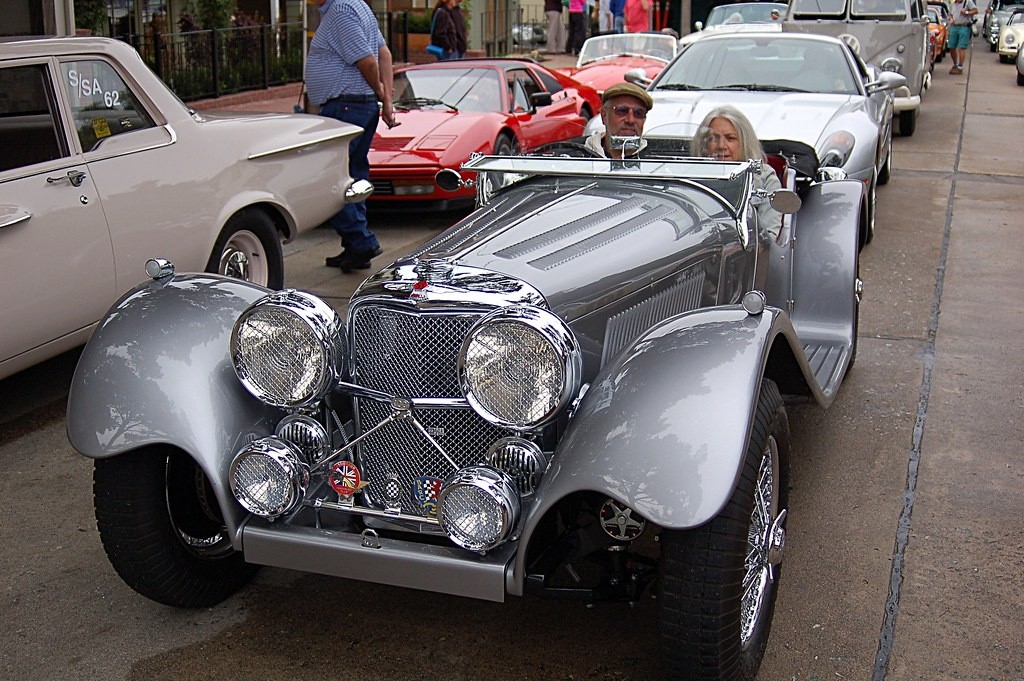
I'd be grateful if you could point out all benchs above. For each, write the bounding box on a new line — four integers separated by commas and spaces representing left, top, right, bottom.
549, 135, 819, 199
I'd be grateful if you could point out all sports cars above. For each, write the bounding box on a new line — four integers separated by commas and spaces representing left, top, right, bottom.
354, 55, 606, 216
553, 32, 679, 106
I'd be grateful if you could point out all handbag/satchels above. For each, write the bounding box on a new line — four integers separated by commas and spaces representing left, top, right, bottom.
426, 45, 444, 57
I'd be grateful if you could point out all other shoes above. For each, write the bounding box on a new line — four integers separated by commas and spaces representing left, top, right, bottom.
949, 66, 963, 74
325, 251, 371, 269
339, 243, 383, 273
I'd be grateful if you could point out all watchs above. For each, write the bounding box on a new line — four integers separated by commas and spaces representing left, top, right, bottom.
965, 11, 969, 15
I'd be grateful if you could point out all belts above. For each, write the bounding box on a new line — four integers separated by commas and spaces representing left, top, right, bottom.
330, 97, 378, 103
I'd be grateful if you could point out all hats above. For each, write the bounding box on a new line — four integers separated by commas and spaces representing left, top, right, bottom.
602, 83, 653, 111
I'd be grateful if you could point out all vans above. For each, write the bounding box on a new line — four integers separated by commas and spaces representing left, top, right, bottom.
779, 0, 940, 136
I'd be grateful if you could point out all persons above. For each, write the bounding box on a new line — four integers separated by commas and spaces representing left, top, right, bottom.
689, 106, 783, 238
650, 27, 680, 62
947, 0, 978, 74
566, 0, 653, 56
544, 0, 566, 52
552, 82, 653, 158
430, 0, 466, 60
305, 0, 401, 272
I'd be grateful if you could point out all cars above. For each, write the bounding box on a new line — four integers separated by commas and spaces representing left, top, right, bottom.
1014, 41, 1024, 86
68, 160, 871, 675
925, 2, 955, 62
0, 39, 372, 382
583, 32, 907, 243
998, 8, 1024, 62
678, 2, 789, 51
982, 0, 1024, 51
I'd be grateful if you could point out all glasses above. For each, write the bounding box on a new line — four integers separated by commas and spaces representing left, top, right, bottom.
603, 106, 646, 119
